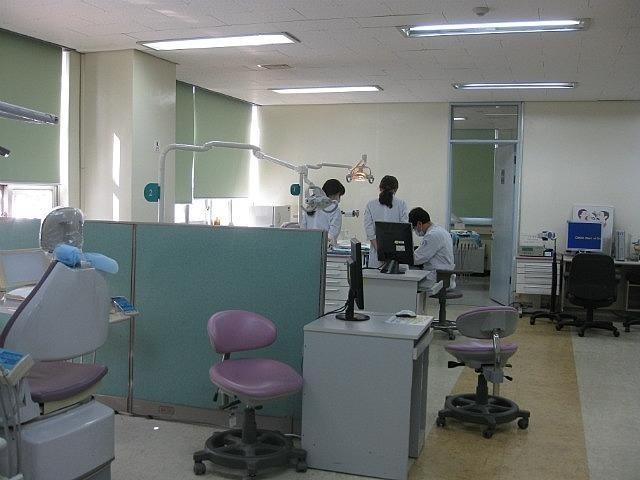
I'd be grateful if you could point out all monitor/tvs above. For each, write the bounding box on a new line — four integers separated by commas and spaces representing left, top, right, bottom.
374, 221, 414, 274
335, 238, 370, 320
566, 219, 602, 252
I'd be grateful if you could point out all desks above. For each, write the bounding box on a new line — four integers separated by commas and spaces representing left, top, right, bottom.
361, 268, 444, 317
302, 309, 434, 479
559, 254, 640, 328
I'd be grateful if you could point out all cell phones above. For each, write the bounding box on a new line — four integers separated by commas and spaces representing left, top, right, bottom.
111, 295, 140, 316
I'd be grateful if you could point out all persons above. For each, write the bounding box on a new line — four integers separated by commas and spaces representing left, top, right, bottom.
577, 208, 589, 223
301, 178, 345, 249
408, 207, 455, 292
596, 209, 612, 252
362, 174, 410, 271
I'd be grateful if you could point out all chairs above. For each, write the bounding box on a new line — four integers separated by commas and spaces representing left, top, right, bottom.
555, 252, 620, 338
193, 307, 309, 480
1, 206, 121, 405
436, 306, 531, 439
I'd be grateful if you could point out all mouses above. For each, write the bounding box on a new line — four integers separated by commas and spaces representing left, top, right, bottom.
396, 310, 416, 318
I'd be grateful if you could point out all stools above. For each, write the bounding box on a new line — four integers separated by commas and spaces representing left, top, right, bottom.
428, 290, 466, 341
